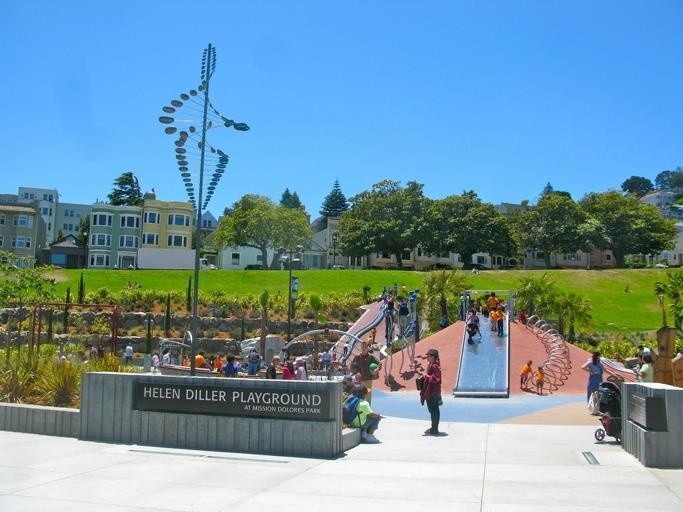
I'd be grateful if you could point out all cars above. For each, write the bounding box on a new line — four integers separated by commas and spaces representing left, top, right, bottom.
333, 265, 345, 270
470, 263, 485, 270
362, 265, 382, 271
431, 263, 450, 269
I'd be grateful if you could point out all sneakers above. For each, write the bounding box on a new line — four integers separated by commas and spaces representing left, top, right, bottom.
362, 432, 379, 443
425, 428, 438, 435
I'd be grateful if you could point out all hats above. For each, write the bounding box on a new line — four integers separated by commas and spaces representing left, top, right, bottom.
293, 359, 305, 365
272, 356, 280, 361
425, 349, 438, 355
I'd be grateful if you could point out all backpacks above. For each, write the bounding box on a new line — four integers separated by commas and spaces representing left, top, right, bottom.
343, 396, 359, 423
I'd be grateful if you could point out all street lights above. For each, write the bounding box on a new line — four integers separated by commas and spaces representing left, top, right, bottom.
277, 244, 305, 361
189, 119, 250, 374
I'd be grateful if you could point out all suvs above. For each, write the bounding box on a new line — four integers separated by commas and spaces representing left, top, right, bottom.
200, 256, 214, 269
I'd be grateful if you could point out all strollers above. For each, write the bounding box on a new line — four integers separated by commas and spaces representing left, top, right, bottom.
593, 373, 625, 443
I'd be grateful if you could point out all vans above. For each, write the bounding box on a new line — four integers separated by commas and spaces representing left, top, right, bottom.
244, 264, 264, 271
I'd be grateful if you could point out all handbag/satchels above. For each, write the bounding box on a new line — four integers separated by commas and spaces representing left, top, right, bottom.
416, 376, 424, 390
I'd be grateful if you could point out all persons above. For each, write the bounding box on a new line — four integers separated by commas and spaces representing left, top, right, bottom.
342, 342, 381, 443
520, 360, 534, 388
122, 341, 135, 367
472, 267, 478, 274
518, 309, 533, 328
534, 367, 544, 394
634, 345, 653, 383
151, 348, 307, 380
55, 342, 105, 363
365, 327, 377, 347
579, 351, 616, 404
308, 341, 351, 370
418, 349, 443, 434
440, 292, 506, 344
377, 289, 408, 336
